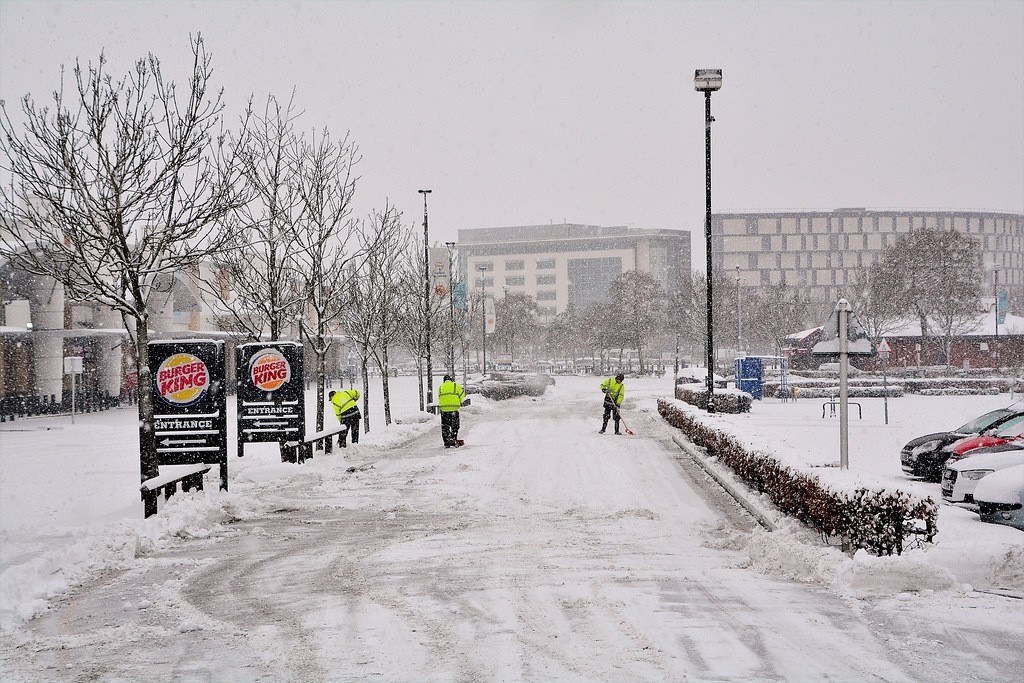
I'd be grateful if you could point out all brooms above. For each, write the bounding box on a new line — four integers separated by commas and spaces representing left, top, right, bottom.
605, 389, 637, 436
454, 435, 464, 445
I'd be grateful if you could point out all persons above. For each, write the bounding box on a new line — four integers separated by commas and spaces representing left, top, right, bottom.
598, 372, 625, 436
328, 390, 361, 447
438, 375, 464, 448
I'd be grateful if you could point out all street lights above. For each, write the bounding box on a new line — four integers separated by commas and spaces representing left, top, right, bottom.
445, 241, 457, 384
477, 267, 487, 378
991, 268, 1004, 370
417, 189, 432, 419
732, 266, 742, 357
694, 66, 723, 399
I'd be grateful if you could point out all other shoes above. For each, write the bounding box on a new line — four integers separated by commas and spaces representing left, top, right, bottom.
598, 430, 623, 435
444, 440, 459, 448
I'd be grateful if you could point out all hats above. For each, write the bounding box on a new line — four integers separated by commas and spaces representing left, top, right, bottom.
444, 375, 452, 381
329, 390, 336, 401
615, 373, 624, 380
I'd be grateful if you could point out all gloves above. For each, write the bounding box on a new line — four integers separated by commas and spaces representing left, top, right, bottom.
605, 389, 610, 394
616, 408, 621, 415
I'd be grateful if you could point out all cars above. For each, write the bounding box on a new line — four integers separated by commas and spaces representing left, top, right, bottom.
898, 397, 1024, 483
965, 464, 1024, 530
949, 411, 1024, 468
940, 442, 1024, 505
